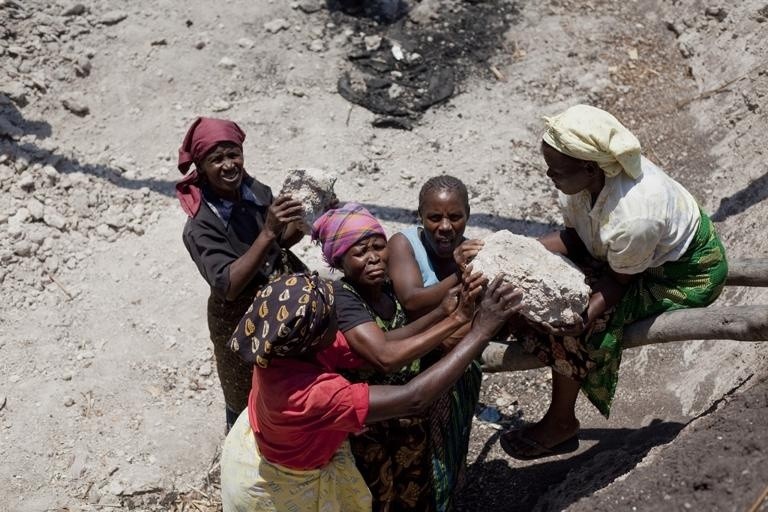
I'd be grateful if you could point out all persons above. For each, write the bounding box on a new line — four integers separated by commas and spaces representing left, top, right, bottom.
173, 116, 311, 432
385, 175, 484, 512
498, 102, 728, 462
216, 270, 525, 511
313, 202, 488, 512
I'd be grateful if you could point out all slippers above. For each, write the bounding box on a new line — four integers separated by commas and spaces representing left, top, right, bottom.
497, 419, 581, 460
